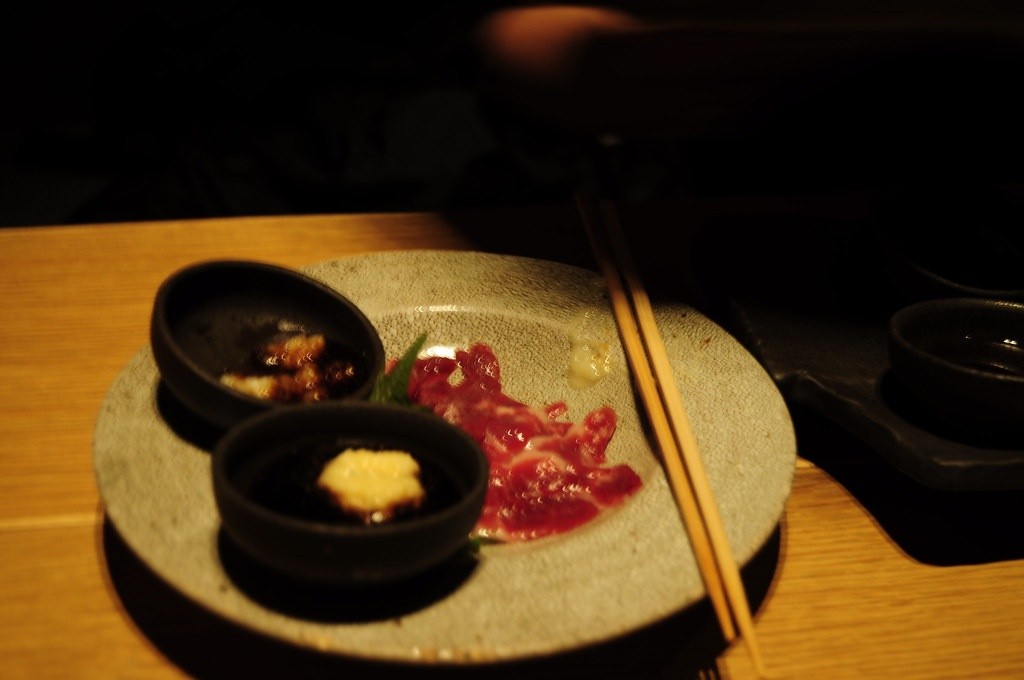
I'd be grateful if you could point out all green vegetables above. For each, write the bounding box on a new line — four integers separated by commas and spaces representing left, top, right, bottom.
365, 325, 435, 412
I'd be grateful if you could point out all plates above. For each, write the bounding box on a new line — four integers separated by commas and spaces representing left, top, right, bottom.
97, 250, 797, 665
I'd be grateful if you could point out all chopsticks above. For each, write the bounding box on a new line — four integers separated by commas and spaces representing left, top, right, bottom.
574, 193, 762, 648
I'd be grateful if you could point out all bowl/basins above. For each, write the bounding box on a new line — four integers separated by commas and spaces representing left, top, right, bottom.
152, 261, 386, 424
872, 191, 1024, 301
888, 297, 1024, 441
213, 402, 486, 579
